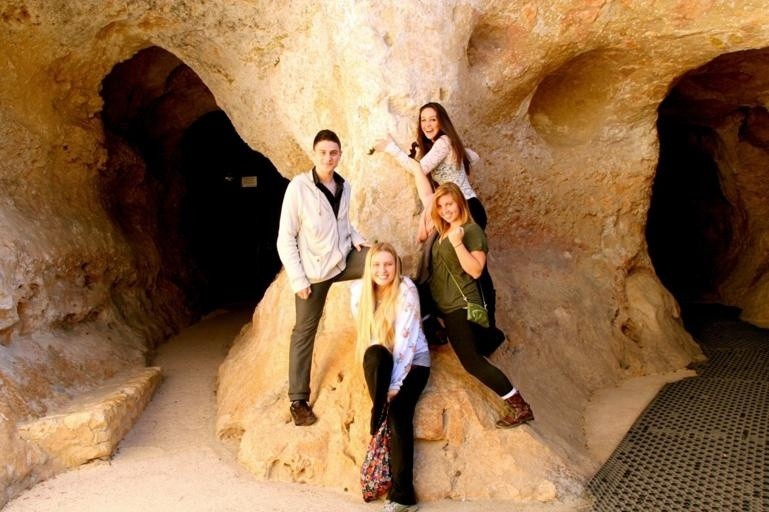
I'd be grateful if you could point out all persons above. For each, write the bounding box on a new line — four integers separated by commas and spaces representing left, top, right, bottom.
277, 129, 402, 425
350, 242, 431, 512
410, 159, 534, 429
375, 102, 496, 326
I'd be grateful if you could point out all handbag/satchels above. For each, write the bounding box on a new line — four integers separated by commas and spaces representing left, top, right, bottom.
359, 398, 394, 504
465, 302, 490, 328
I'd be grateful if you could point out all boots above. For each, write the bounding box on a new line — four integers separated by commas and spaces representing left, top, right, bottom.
422, 314, 449, 345
494, 390, 534, 430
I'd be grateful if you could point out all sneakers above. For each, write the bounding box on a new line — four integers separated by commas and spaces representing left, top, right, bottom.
288, 400, 319, 427
376, 497, 419, 512
